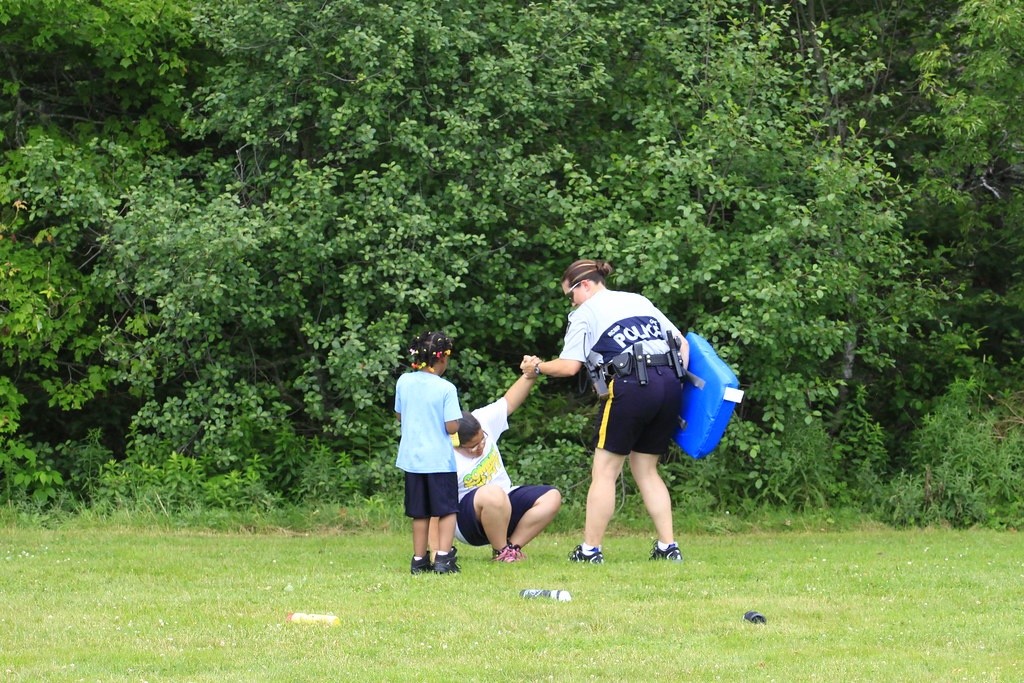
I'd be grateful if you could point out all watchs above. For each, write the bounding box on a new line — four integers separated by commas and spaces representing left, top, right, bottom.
533, 361, 542, 377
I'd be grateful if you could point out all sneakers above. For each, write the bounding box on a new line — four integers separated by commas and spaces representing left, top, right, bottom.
491, 538, 527, 564
649, 539, 682, 561
567, 544, 604, 565
410, 550, 434, 575
433, 546, 461, 575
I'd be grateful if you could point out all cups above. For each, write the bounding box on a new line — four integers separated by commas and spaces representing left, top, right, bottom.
451, 433, 459, 448
284, 613, 339, 626
519, 588, 569, 603
744, 610, 766, 623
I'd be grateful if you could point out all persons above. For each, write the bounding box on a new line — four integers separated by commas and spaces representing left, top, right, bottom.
519, 259, 688, 564
449, 354, 562, 565
393, 330, 463, 574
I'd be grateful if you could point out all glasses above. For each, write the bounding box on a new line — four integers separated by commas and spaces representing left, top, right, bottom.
460, 429, 488, 455
565, 282, 581, 302
